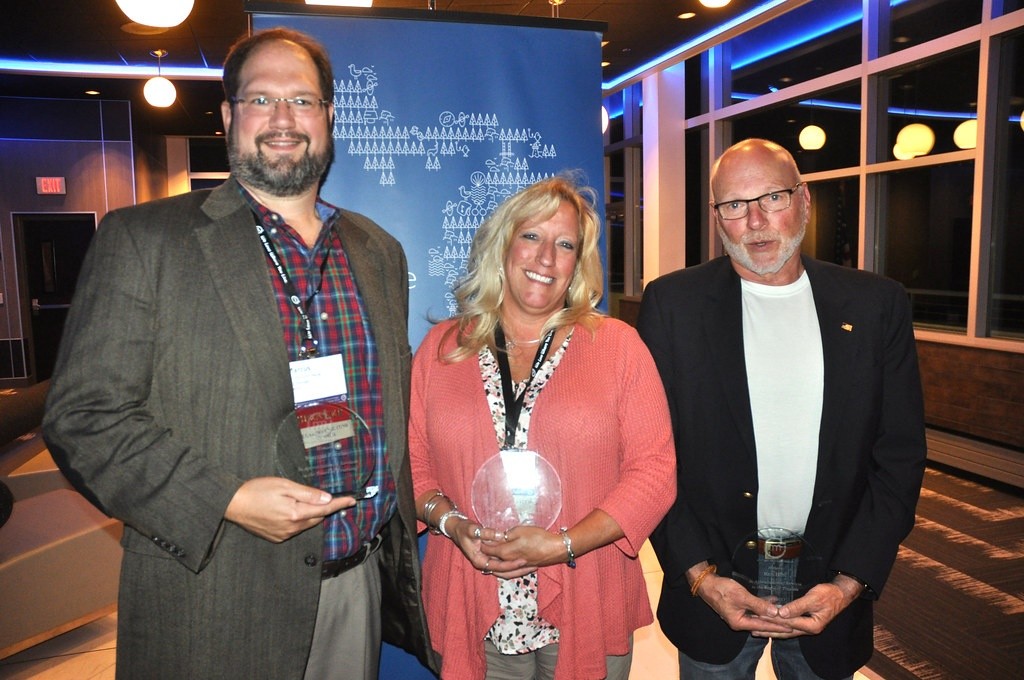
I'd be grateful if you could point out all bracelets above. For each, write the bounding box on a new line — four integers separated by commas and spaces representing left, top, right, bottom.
439, 511, 468, 539
691, 565, 717, 598
423, 492, 457, 536
557, 532, 576, 569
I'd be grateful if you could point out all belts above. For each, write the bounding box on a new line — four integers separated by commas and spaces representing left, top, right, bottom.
321, 536, 379, 580
758, 539, 802, 560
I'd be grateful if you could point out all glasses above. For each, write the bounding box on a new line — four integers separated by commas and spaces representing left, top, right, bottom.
712, 181, 804, 220
229, 96, 330, 117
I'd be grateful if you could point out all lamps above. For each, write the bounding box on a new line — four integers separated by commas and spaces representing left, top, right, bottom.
895, 82, 936, 156
798, 98, 827, 151
953, 119, 977, 149
892, 82, 913, 159
143, 48, 177, 108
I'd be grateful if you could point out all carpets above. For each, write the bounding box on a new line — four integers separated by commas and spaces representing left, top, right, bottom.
856, 458, 1024, 680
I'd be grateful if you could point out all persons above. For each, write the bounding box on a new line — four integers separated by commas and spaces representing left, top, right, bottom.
637, 138, 929, 680
404, 176, 678, 680
39, 27, 425, 680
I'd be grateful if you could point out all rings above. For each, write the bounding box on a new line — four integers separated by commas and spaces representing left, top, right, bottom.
485, 559, 491, 569
481, 569, 494, 575
503, 532, 508, 541
